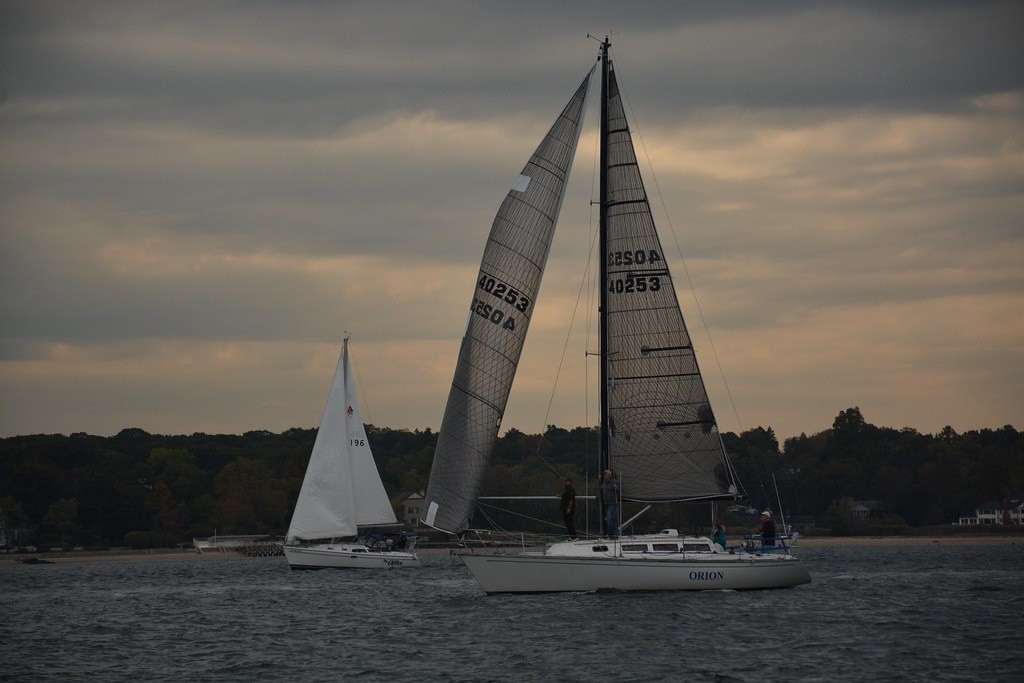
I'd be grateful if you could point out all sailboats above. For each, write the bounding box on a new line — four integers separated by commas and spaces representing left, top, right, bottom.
419, 28, 813, 593
282, 328, 417, 570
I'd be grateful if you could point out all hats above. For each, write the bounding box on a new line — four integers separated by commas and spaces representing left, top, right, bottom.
602, 470, 612, 475
760, 511, 770, 519
564, 478, 573, 483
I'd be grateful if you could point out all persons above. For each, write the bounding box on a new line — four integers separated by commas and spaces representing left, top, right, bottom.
556, 479, 579, 542
712, 520, 727, 550
601, 469, 619, 540
752, 511, 776, 554
